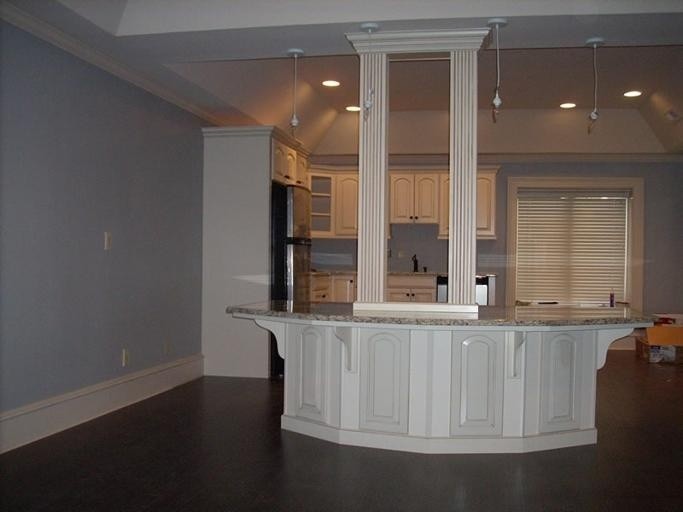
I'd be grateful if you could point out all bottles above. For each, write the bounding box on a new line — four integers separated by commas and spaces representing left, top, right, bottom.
610, 288, 614, 307
410, 254, 418, 272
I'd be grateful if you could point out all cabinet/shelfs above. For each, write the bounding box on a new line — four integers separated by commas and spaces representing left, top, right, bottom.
310, 275, 331, 303
331, 274, 358, 302
389, 166, 440, 225
304, 164, 359, 240
202, 125, 311, 188
386, 274, 437, 304
438, 165, 502, 239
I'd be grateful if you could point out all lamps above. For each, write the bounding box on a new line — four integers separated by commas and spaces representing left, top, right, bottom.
487, 18, 509, 115
286, 49, 306, 127
585, 38, 608, 122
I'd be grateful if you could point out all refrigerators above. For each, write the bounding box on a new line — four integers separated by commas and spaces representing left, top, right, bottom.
271, 184, 311, 375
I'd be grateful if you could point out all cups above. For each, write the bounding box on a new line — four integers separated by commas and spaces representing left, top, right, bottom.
423, 264, 427, 272
